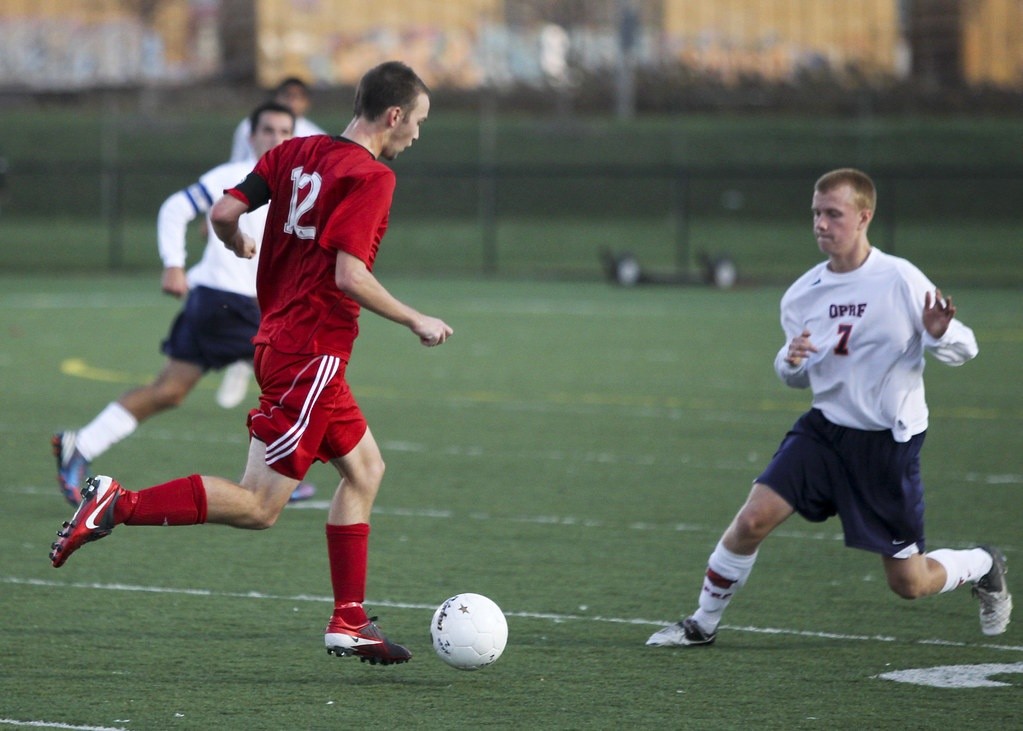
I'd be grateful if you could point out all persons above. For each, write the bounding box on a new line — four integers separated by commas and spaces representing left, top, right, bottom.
644, 168, 1012, 647
50, 61, 453, 666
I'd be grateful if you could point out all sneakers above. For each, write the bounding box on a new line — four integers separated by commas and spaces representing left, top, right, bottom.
214, 359, 252, 409
290, 482, 314, 502
51, 429, 92, 506
325, 613, 412, 666
644, 616, 717, 646
48, 474, 127, 568
969, 544, 1013, 635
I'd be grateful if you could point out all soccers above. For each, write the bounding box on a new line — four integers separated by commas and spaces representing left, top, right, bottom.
429, 592, 510, 672
